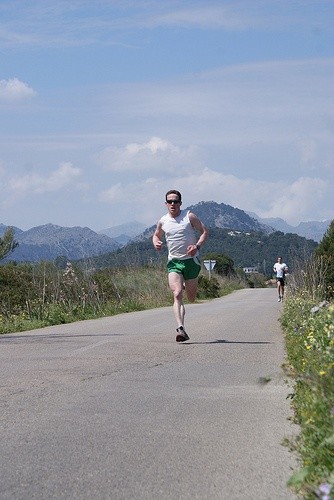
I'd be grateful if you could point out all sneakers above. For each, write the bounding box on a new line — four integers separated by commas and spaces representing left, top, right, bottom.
176, 326, 189, 342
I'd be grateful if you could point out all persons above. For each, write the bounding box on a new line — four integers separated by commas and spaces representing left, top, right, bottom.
153, 189, 208, 342
273, 256, 289, 302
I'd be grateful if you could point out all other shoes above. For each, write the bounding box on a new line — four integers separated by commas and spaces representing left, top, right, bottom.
277, 296, 284, 302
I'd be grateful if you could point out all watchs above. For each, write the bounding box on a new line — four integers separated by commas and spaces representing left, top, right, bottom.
195, 244, 200, 250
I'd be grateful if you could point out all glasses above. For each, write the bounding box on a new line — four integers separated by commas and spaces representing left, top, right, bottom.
166, 200, 179, 204
